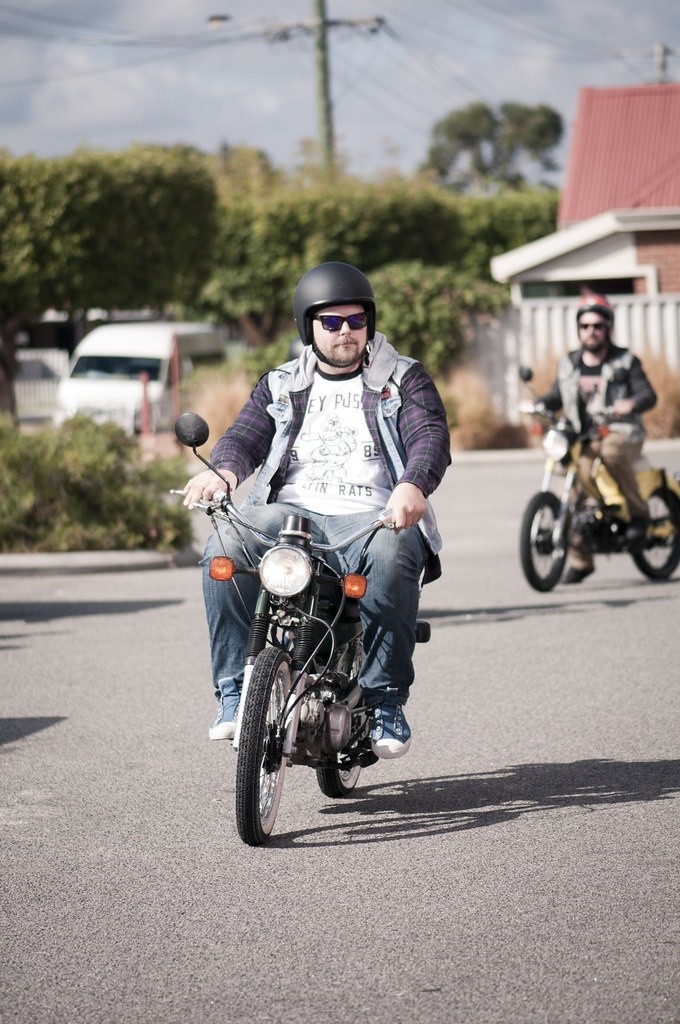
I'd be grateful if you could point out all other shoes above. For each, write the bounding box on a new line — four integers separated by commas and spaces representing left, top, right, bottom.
626, 516, 649, 542
561, 566, 594, 584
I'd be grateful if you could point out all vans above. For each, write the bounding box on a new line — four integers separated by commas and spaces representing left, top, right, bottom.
53, 320, 227, 437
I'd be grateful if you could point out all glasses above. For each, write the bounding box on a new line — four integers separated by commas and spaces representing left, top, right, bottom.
579, 323, 606, 329
313, 311, 368, 332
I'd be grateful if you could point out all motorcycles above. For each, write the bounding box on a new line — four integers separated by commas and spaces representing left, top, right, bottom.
167, 412, 431, 844
511, 364, 680, 594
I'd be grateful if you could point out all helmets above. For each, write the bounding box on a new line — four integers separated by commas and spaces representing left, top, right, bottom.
577, 293, 614, 329
293, 262, 376, 346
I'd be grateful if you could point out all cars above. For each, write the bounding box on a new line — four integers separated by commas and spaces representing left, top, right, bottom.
13, 348, 69, 420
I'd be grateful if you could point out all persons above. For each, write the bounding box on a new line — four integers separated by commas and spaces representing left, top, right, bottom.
523, 304, 658, 584
183, 263, 452, 758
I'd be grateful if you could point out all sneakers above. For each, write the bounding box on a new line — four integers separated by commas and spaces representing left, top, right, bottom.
209, 677, 242, 741
371, 702, 411, 759
